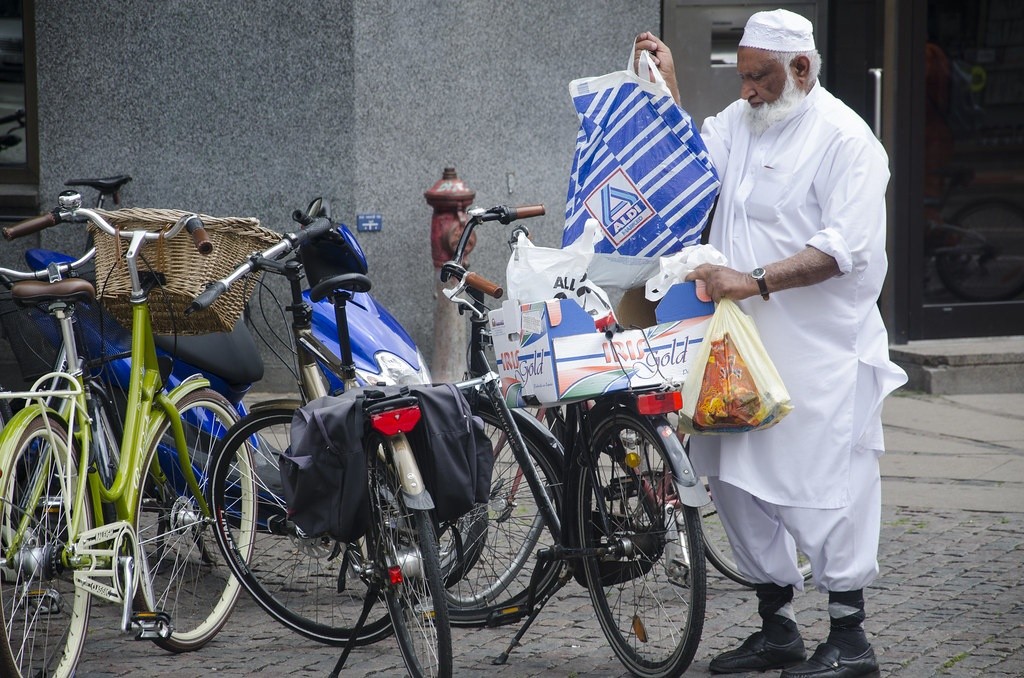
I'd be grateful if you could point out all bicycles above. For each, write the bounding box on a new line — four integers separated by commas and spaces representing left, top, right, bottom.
367, 201, 711, 677
182, 194, 454, 678
0, 176, 258, 678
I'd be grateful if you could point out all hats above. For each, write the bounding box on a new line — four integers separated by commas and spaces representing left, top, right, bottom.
739, 9, 817, 53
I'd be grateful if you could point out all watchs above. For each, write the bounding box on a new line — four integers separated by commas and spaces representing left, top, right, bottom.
751, 267, 770, 302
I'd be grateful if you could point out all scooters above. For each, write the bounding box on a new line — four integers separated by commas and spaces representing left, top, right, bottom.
1, 196, 490, 598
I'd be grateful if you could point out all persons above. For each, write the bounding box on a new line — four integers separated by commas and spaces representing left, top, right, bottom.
634, 7, 907, 678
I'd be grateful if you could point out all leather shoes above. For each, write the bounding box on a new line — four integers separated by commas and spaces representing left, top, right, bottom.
710, 633, 808, 673
780, 643, 880, 678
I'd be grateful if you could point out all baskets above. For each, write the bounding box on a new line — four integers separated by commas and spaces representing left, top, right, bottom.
84, 208, 284, 335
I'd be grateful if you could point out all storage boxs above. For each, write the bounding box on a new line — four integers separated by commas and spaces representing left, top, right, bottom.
488, 279, 716, 412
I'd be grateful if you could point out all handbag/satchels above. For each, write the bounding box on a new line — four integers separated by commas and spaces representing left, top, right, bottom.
566, 33, 720, 289
280, 384, 494, 538
